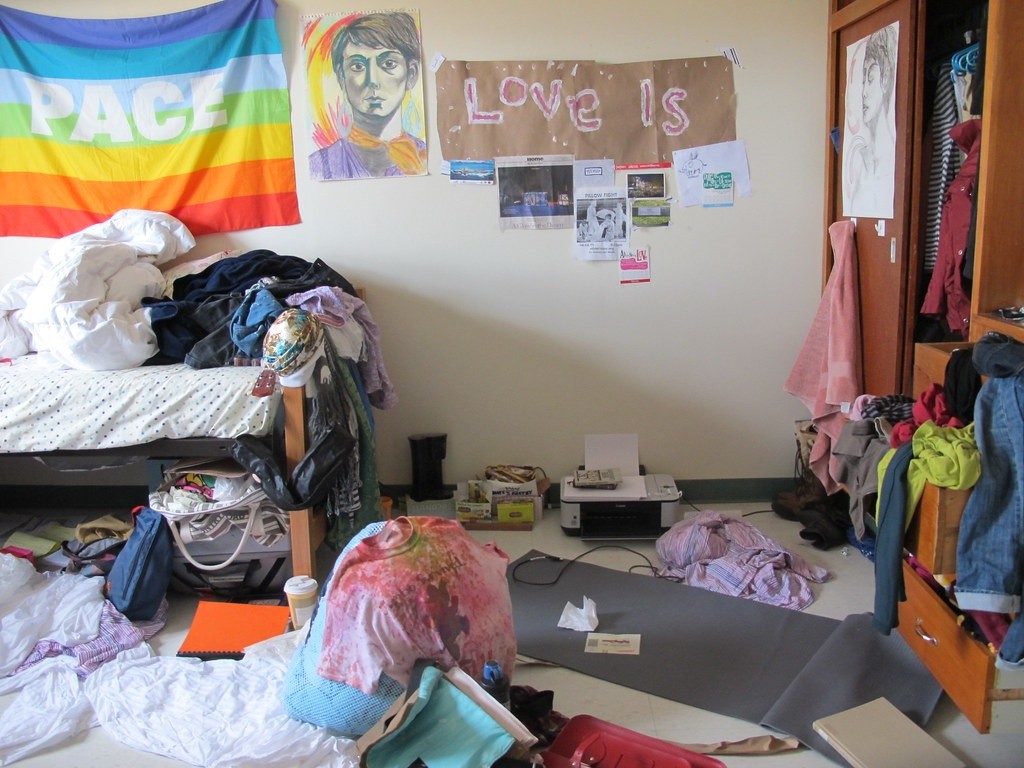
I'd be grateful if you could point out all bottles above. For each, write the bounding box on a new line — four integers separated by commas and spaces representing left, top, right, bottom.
479, 660, 511, 711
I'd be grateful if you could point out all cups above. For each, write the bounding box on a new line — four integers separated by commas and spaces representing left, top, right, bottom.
283, 575, 319, 631
380, 496, 393, 518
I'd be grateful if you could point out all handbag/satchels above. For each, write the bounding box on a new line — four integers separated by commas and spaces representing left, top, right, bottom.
104, 508, 180, 621
150, 458, 268, 571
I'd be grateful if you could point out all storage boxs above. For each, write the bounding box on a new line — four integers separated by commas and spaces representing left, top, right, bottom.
455, 473, 543, 531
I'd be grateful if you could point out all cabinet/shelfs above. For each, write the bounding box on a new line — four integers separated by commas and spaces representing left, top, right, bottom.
820, 1, 1024, 737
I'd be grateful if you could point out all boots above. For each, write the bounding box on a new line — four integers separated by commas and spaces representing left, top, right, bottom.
772, 419, 824, 520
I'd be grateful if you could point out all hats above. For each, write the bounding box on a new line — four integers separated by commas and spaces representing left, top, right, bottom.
262, 309, 323, 377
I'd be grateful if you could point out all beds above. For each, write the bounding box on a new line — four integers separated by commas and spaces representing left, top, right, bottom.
0, 289, 368, 579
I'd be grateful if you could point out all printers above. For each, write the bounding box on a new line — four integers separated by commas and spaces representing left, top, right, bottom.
559, 475, 679, 543
